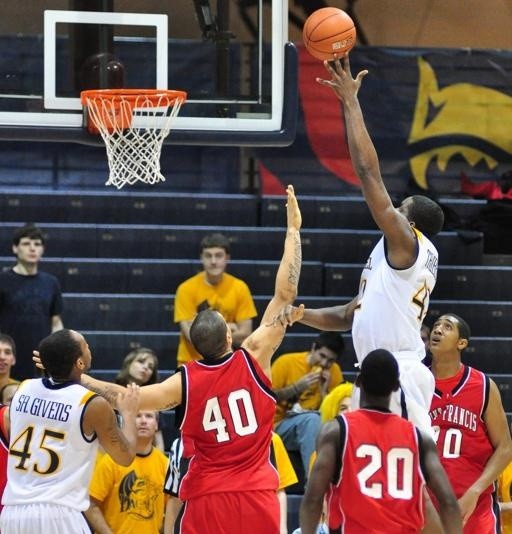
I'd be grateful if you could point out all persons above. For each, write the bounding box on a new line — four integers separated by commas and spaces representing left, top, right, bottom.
30, 182, 303, 534
296, 348, 461, 534
419, 323, 432, 373
172, 231, 257, 366
262, 51, 447, 534
424, 313, 512, 534
0, 222, 65, 382
0, 327, 356, 534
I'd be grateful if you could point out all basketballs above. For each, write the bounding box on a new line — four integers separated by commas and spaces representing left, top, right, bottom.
303, 7, 356, 61
